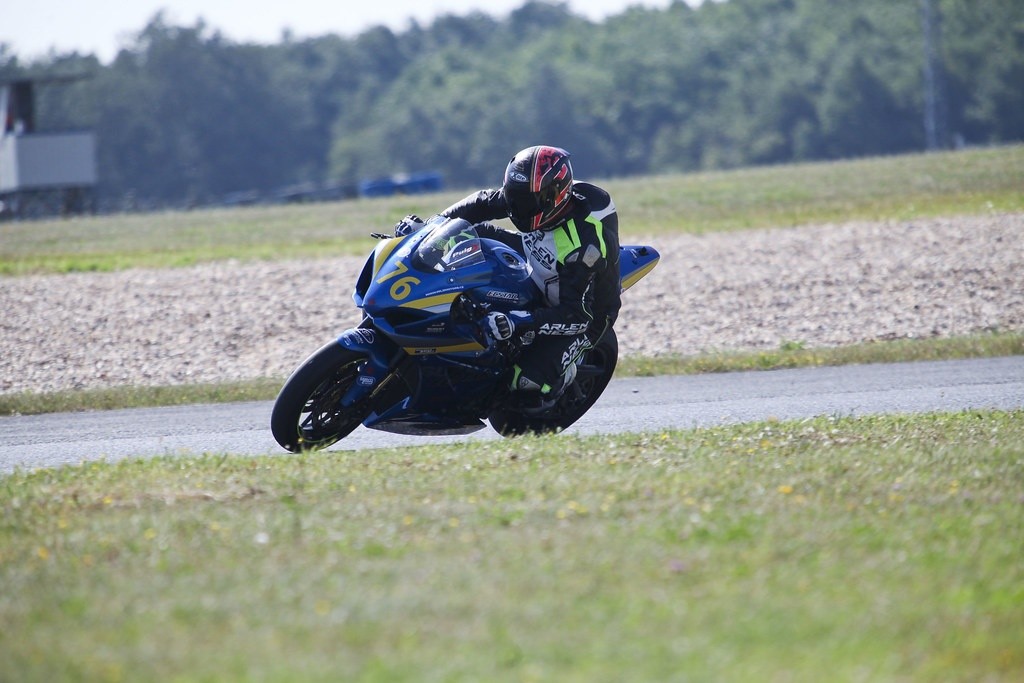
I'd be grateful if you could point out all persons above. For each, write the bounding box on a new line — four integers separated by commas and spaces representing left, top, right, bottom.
395, 144, 620, 418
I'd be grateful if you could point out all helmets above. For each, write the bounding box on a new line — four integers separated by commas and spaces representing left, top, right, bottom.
504, 145, 572, 232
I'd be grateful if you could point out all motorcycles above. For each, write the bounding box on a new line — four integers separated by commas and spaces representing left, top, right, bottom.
270, 219, 659, 454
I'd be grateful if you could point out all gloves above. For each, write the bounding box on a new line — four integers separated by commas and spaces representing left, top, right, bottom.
394, 215, 426, 237
478, 309, 536, 345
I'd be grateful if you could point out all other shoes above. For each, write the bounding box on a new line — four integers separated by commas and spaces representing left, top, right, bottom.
525, 360, 577, 413
